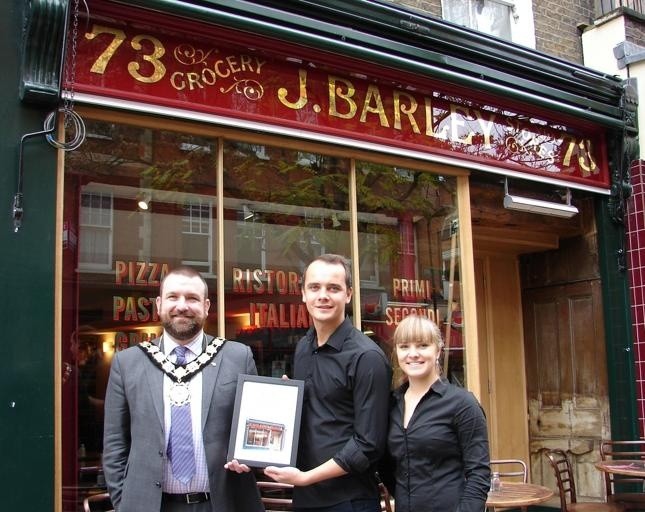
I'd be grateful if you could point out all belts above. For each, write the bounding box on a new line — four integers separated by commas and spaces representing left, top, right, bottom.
161, 492, 210, 505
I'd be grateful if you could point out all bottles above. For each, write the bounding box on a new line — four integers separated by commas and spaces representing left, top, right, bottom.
491, 471, 500, 492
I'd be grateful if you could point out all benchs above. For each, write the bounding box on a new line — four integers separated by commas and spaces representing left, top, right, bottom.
81, 465, 110, 512
257, 481, 294, 509
377, 481, 392, 511
490, 459, 528, 483
600, 442, 644, 503
545, 449, 626, 510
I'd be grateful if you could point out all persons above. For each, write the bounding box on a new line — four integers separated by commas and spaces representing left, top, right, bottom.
100, 265, 270, 512
373, 312, 493, 512
64, 322, 99, 491
263, 252, 394, 512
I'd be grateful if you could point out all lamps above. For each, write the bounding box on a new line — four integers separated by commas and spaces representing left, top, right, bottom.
235, 203, 343, 228
503, 177, 578, 219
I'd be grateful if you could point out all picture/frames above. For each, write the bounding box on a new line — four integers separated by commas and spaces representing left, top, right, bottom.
226, 373, 305, 470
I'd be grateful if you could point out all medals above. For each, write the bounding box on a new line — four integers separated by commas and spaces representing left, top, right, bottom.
168, 382, 192, 407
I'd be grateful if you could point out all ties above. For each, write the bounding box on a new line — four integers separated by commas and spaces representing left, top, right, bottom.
167, 345, 196, 487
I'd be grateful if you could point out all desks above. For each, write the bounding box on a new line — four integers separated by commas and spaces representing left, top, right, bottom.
594, 460, 644, 477
484, 482, 553, 511
